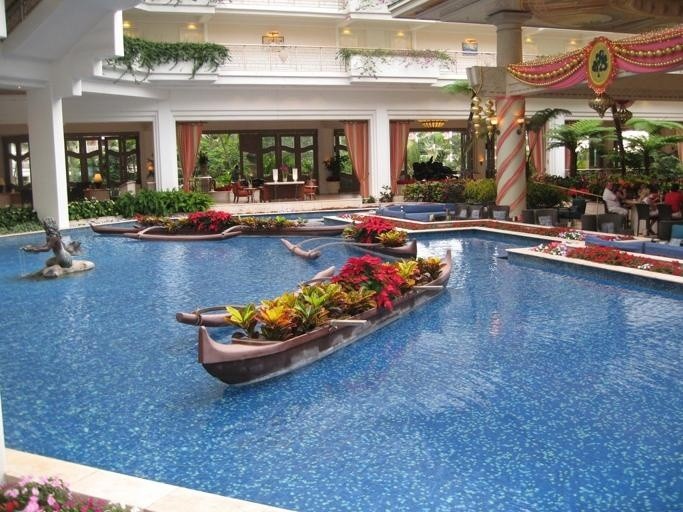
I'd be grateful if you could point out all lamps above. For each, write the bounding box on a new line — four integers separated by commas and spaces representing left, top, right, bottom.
418, 118, 447, 130
588, 86, 614, 118
489, 116, 499, 133
144, 158, 154, 178
613, 97, 633, 125
91, 174, 102, 189
515, 119, 525, 135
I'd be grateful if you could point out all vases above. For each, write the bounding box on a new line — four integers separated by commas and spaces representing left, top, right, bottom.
283, 176, 288, 183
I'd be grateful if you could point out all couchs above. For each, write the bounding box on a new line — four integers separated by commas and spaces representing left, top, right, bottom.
384, 202, 454, 222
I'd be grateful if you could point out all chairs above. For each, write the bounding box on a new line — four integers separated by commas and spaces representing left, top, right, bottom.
450, 198, 632, 235
635, 203, 660, 236
229, 178, 318, 202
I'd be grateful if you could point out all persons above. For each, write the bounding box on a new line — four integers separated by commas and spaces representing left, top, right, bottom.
232, 165, 240, 182
601, 173, 683, 237
18, 217, 82, 267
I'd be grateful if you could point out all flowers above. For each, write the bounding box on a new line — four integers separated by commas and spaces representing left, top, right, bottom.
280, 164, 287, 176
189, 210, 231, 233
330, 254, 404, 311
355, 215, 391, 244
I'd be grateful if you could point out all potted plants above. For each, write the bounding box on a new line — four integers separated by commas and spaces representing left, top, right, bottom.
323, 155, 349, 194
197, 150, 209, 175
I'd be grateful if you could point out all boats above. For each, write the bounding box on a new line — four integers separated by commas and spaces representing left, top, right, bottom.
342, 229, 417, 262
88, 220, 356, 237
506, 242, 683, 295
198, 248, 453, 389
